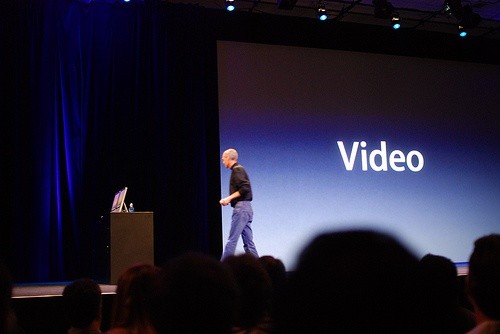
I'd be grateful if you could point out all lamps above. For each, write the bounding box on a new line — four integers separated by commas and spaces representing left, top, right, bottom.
458, 25, 467, 38
315, 4, 327, 21
392, 18, 401, 29
225, 0, 235, 12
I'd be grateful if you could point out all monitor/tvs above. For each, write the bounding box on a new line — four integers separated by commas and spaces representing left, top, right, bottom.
111, 186, 127, 212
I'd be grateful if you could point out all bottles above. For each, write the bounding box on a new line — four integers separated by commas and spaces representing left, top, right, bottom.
129, 203, 134, 212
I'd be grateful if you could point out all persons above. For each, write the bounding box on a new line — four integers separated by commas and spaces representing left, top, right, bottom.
0, 230, 500, 334
220, 149, 258, 262
